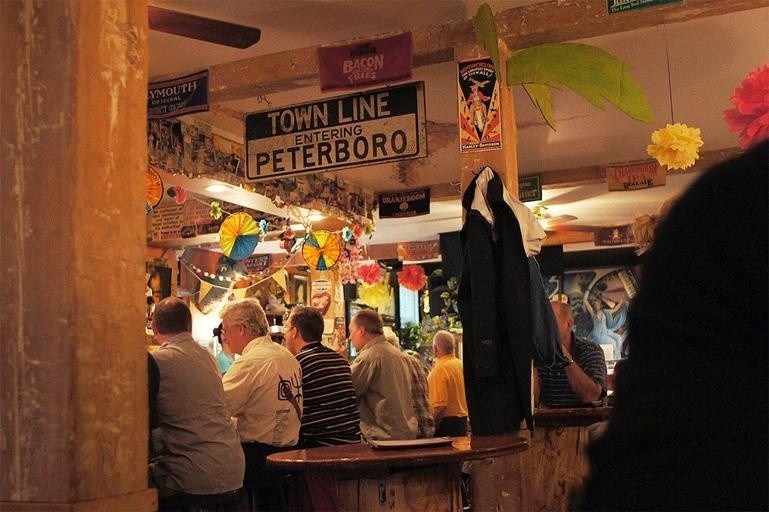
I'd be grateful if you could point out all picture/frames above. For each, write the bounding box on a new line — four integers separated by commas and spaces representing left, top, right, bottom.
289, 272, 312, 307
144, 256, 180, 346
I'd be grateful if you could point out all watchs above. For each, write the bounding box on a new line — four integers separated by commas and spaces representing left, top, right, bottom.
565, 357, 574, 366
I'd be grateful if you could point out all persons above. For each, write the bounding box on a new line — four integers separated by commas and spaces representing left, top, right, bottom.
582, 286, 630, 359
463, 84, 492, 126
146, 296, 246, 500
213, 301, 303, 480
535, 302, 609, 408
285, 306, 362, 448
349, 311, 418, 441
382, 325, 436, 439
426, 331, 468, 438
